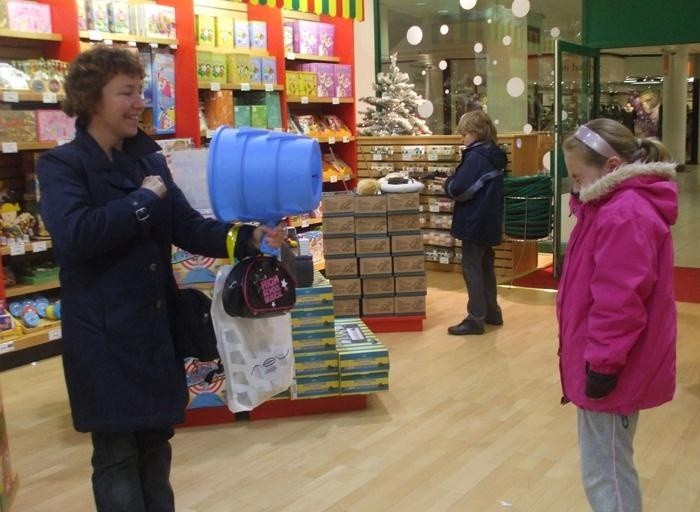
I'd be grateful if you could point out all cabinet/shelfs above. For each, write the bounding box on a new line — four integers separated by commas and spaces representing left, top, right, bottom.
0, 0, 363, 369
357, 131, 556, 284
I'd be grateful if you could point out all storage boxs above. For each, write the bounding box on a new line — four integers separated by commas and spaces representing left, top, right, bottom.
273, 187, 428, 400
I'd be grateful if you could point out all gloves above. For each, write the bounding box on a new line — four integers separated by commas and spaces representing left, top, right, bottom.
585, 369, 618, 399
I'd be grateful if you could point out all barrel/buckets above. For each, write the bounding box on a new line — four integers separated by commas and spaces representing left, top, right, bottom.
206, 123, 325, 220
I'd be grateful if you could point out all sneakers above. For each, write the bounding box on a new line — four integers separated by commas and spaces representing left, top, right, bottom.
449, 318, 484, 334
485, 319, 503, 325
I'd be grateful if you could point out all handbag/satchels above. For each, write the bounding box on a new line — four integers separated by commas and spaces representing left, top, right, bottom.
222, 253, 296, 318
169, 288, 218, 363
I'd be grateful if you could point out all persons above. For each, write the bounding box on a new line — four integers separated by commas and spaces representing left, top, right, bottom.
556, 118, 678, 512
36, 48, 286, 512
433, 110, 507, 334
